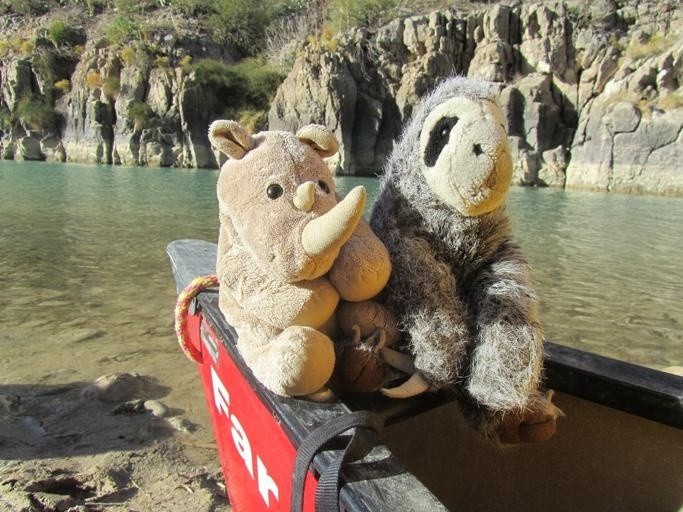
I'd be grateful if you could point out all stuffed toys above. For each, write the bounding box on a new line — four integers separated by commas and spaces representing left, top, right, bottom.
369, 74, 555, 444
207, 119, 400, 403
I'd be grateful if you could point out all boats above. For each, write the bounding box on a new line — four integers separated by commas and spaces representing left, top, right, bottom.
166, 239, 682, 512
